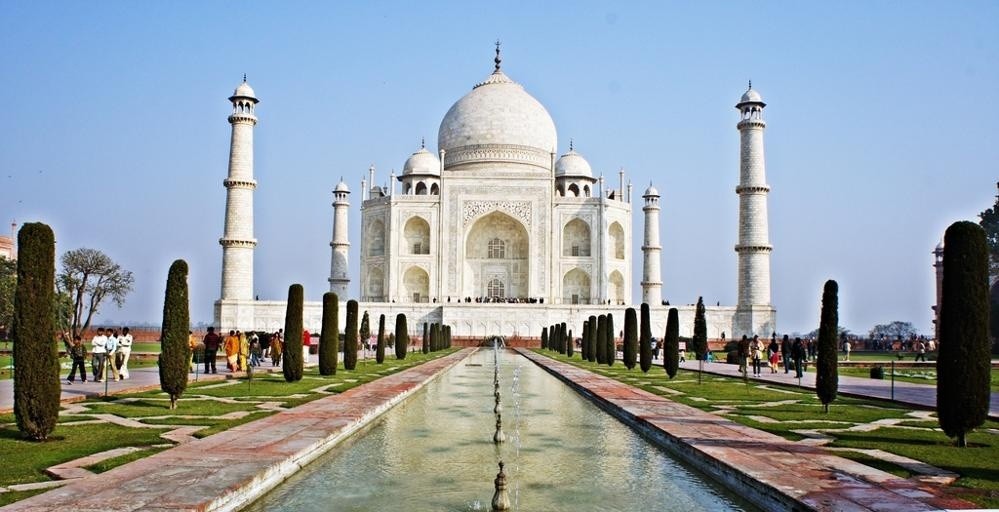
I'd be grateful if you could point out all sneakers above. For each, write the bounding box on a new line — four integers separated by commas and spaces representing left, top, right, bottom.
793, 375, 800, 378
97, 378, 105, 382
771, 371, 775, 374
81, 379, 88, 385
774, 369, 777, 374
189, 370, 195, 373
203, 370, 209, 374
753, 373, 757, 377
118, 373, 124, 380
758, 373, 761, 377
114, 376, 120, 383
66, 379, 73, 386
212, 370, 217, 374
738, 369, 743, 373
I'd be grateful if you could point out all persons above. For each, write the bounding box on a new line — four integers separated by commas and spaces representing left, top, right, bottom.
678, 349, 685, 363
622, 302, 625, 305
879, 337, 936, 361
698, 296, 703, 303
255, 294, 259, 301
303, 328, 310, 364
748, 335, 764, 377
603, 300, 606, 304
464, 296, 543, 303
716, 301, 720, 306
656, 339, 664, 359
66, 335, 88, 384
412, 335, 416, 346
416, 335, 421, 347
575, 338, 582, 348
791, 337, 806, 378
707, 348, 713, 363
662, 300, 669, 305
721, 331, 725, 341
801, 339, 851, 362
651, 337, 658, 360
365, 333, 377, 351
608, 299, 611, 304
203, 327, 219, 374
458, 299, 461, 303
447, 295, 450, 303
217, 332, 223, 351
737, 334, 750, 372
224, 329, 284, 373
392, 299, 395, 303
432, 297, 436, 303
781, 335, 791, 373
767, 339, 779, 374
615, 341, 623, 357
90, 326, 133, 383
188, 331, 197, 374
